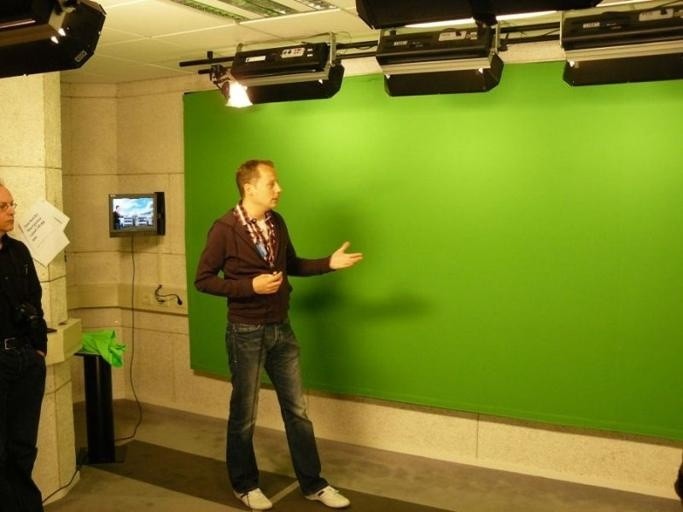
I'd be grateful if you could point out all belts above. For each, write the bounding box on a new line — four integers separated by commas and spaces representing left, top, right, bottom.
1, 337, 19, 352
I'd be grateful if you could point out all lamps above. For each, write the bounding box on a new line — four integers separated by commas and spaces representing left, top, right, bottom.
206, 63, 256, 109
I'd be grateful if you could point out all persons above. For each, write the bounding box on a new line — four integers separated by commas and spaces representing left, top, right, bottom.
194, 157, 364, 511
0, 184, 50, 510
113, 205, 124, 228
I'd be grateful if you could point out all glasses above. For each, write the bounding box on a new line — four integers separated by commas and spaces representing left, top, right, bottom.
0, 201, 18, 212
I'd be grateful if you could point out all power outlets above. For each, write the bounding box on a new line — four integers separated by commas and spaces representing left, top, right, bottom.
152, 292, 168, 308
174, 292, 187, 308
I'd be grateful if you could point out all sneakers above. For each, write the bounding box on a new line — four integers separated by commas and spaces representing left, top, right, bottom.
303, 484, 352, 510
231, 487, 274, 511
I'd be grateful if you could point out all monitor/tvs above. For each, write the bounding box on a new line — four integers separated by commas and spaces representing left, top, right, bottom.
108, 193, 165, 236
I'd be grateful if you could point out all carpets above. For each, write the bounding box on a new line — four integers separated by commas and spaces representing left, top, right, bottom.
270, 473, 457, 512
88, 438, 297, 511
